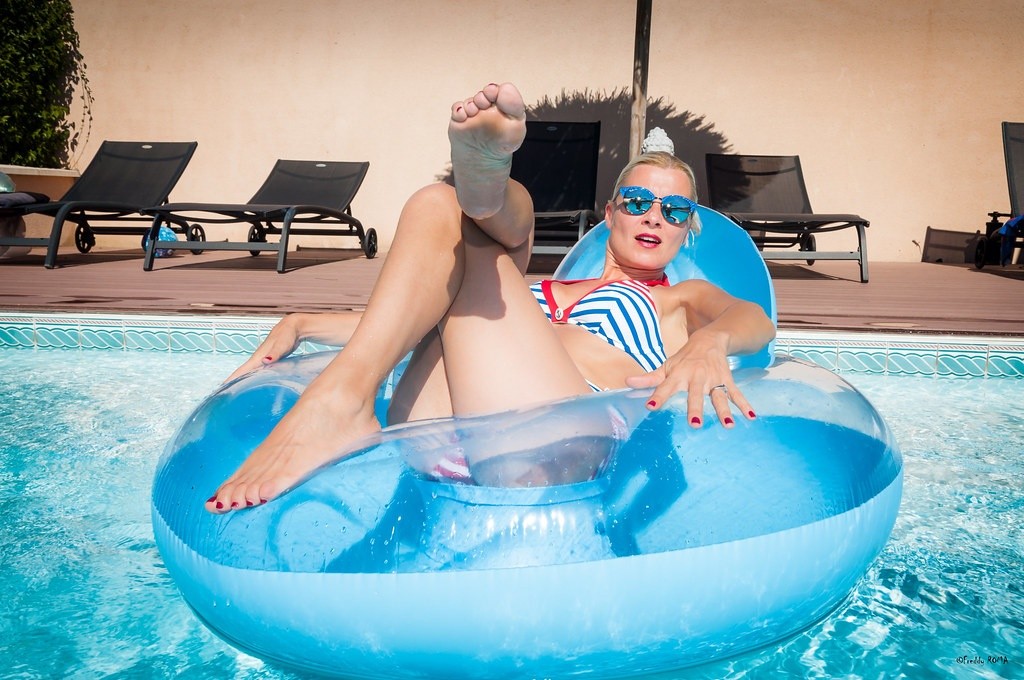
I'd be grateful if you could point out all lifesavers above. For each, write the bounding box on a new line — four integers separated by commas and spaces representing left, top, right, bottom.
146, 351, 907, 680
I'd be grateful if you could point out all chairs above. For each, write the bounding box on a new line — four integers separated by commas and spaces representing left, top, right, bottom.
0, 140, 206, 270
705, 152, 872, 283
139, 159, 378, 273
973, 121, 1024, 270
509, 119, 605, 255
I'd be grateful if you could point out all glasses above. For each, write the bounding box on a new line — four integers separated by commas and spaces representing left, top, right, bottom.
612, 184, 698, 225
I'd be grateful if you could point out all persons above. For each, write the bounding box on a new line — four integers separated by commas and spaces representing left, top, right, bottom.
202, 81, 774, 517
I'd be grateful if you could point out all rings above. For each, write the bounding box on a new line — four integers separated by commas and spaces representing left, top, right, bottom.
707, 385, 729, 397
